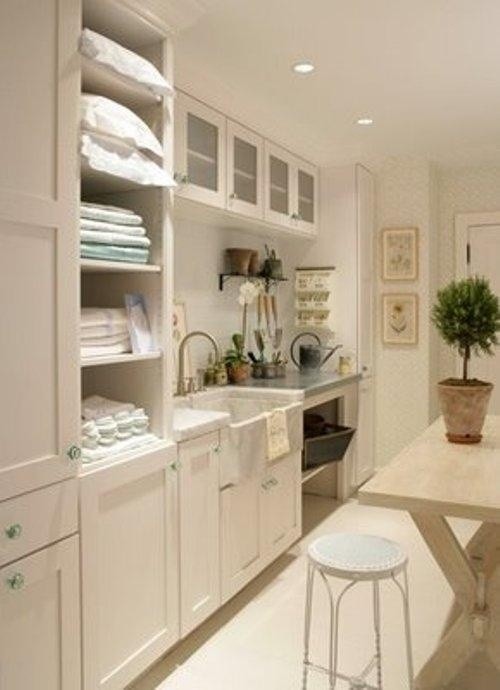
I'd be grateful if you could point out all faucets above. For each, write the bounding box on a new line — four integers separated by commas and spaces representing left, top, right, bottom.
176, 329, 220, 394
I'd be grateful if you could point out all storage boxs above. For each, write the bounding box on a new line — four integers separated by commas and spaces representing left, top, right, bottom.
302, 424, 356, 469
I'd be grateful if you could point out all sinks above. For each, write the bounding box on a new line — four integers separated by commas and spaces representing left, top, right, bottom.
187, 395, 304, 489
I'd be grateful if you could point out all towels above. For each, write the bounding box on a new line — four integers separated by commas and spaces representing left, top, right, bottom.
267, 409, 290, 460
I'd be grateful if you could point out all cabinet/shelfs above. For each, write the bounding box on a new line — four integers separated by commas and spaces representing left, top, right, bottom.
175, 86, 264, 229
293, 163, 374, 496
80, 446, 179, 690
0, 0, 81, 690
78, 0, 174, 465
178, 430, 221, 641
265, 138, 322, 240
221, 413, 302, 605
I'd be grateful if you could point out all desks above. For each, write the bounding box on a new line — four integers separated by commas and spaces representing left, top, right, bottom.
358, 412, 499, 689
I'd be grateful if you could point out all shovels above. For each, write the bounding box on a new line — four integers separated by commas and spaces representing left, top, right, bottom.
270, 295, 283, 349
253, 294, 266, 353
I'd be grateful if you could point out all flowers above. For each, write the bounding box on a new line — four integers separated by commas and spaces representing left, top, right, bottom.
223, 282, 261, 366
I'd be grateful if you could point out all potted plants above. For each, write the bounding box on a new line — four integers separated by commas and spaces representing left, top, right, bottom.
429, 276, 500, 442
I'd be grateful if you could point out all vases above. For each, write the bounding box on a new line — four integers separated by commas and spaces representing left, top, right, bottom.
227, 362, 247, 382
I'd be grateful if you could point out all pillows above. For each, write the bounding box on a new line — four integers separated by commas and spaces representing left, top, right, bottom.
81, 92, 162, 157
81, 129, 175, 186
81, 28, 173, 96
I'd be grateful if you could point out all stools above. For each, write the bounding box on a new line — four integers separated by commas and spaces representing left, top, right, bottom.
303, 534, 414, 690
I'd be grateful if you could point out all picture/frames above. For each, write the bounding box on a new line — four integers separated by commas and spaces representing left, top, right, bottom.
380, 227, 419, 282
381, 294, 418, 343
